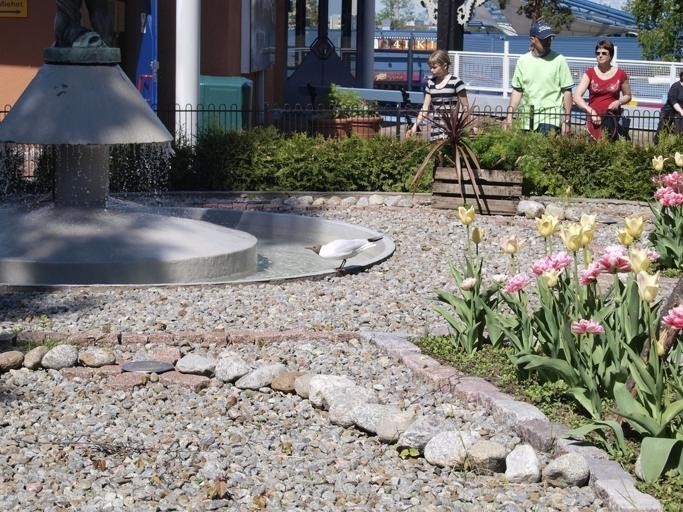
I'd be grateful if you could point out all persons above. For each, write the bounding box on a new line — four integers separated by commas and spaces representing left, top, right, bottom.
652, 68, 682, 146
570, 38, 631, 141
403, 49, 471, 143
501, 22, 574, 137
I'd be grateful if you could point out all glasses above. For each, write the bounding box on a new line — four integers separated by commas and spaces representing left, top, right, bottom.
596, 52, 607, 55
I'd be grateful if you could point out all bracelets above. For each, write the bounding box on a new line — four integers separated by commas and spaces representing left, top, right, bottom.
617, 99, 621, 105
562, 118, 569, 124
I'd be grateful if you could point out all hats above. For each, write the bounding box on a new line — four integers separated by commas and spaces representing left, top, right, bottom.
530, 24, 555, 39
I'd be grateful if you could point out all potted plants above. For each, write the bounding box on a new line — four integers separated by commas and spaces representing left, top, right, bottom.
312, 84, 352, 138
351, 100, 382, 138
